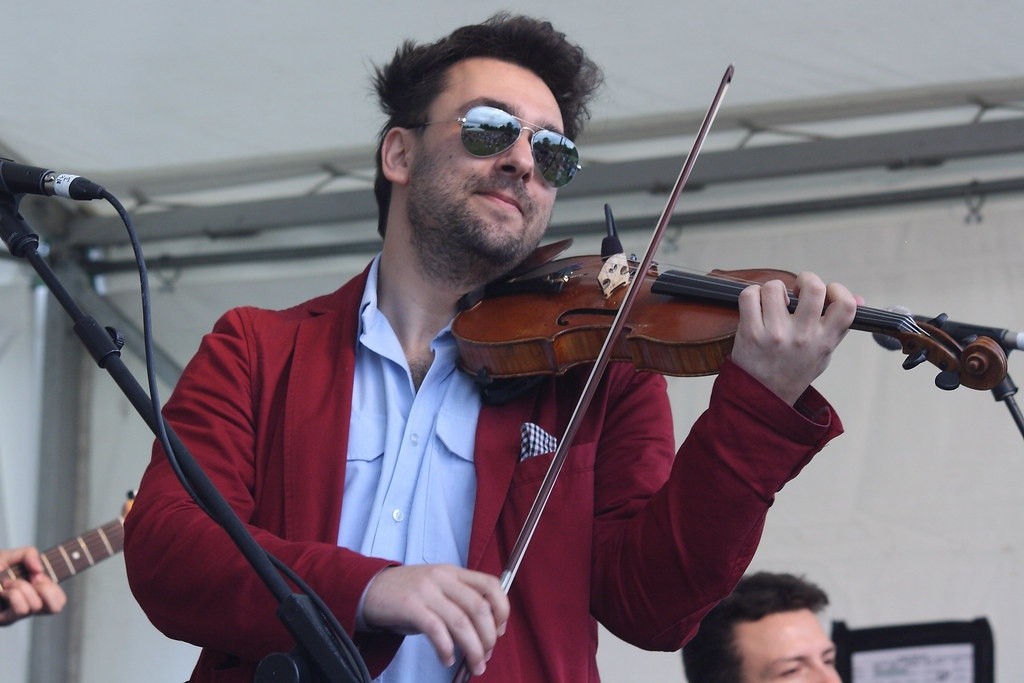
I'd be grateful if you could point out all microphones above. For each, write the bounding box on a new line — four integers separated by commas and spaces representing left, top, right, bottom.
600, 203, 625, 265
0, 159, 105, 201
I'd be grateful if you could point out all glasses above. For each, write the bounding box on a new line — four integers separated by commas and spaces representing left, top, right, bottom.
417, 106, 581, 189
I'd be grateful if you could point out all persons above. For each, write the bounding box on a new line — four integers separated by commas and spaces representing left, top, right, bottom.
122, 17, 863, 683
682, 572, 846, 683
0, 546, 67, 627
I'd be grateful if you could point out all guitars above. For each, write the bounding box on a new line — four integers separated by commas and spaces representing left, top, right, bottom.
0, 489, 141, 614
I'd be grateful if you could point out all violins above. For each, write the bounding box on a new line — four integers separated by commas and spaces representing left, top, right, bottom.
452, 254, 1008, 408
